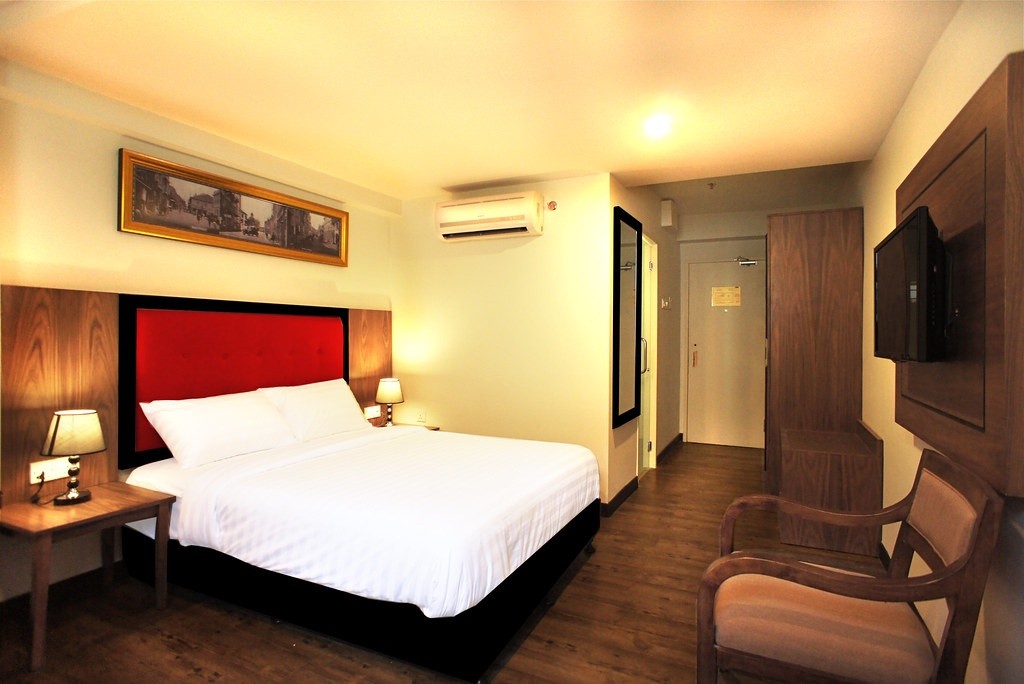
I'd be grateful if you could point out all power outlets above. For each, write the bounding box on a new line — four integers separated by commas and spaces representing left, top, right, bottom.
416, 410, 427, 422
29, 456, 80, 485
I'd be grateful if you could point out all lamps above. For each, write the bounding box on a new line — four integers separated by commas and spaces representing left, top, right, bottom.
41, 409, 107, 504
374, 377, 404, 427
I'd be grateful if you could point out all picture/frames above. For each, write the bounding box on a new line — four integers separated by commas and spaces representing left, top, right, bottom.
117, 148, 349, 267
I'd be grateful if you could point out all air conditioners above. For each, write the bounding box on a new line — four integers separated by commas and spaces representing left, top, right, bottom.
434, 190, 543, 244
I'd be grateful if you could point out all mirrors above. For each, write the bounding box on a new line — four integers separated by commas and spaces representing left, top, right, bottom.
613, 205, 642, 429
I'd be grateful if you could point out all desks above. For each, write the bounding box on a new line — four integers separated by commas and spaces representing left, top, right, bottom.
0, 480, 176, 671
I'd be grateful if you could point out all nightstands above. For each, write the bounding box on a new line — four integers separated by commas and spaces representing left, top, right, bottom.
378, 422, 440, 431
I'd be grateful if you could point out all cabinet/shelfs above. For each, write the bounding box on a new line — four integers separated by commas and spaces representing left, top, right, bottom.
764, 206, 865, 476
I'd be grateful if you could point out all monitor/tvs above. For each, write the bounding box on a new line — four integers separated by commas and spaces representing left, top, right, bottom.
873, 205, 949, 363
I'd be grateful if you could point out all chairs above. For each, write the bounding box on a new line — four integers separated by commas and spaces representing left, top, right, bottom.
696, 448, 1005, 684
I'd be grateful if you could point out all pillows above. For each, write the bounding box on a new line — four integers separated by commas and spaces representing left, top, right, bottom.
138, 391, 269, 466
258, 377, 375, 448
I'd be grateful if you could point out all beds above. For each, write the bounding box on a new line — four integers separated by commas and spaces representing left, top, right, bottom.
122, 428, 602, 683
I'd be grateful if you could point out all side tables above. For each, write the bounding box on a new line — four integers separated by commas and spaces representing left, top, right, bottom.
779, 419, 884, 556
